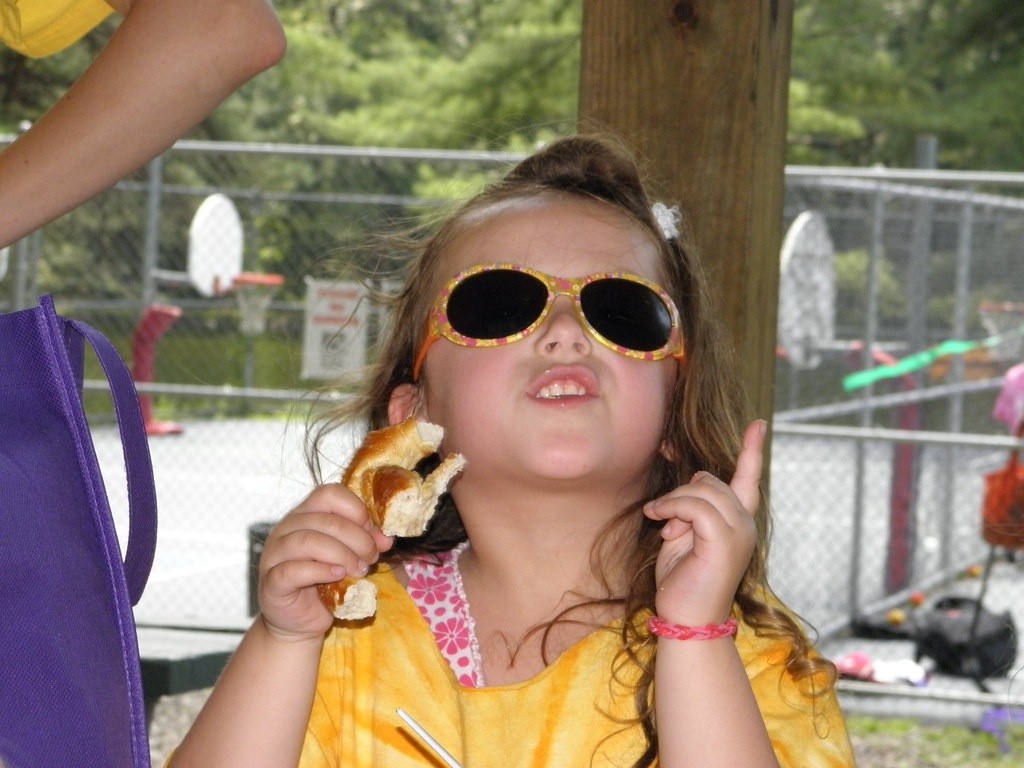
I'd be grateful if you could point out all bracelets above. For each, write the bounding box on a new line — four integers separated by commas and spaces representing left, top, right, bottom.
640, 615, 738, 639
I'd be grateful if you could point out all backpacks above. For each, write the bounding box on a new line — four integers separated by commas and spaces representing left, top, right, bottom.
917, 596, 1017, 677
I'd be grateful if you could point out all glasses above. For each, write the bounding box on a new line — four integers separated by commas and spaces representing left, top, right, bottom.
414, 262, 684, 379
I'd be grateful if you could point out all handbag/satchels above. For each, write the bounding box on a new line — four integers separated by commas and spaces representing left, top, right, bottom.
0, 294, 159, 768
982, 421, 1024, 547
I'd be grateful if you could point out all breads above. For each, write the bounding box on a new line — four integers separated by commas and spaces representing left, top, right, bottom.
313, 421, 467, 622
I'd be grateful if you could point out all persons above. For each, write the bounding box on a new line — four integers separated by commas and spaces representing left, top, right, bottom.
162, 137, 852, 768
0, 0, 288, 254
